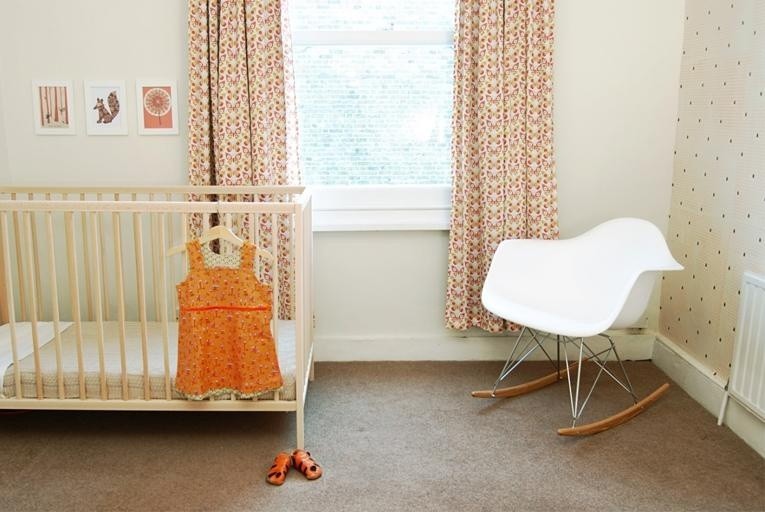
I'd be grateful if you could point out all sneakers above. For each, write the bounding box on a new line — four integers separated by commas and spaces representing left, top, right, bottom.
291, 448, 323, 480
266, 452, 291, 486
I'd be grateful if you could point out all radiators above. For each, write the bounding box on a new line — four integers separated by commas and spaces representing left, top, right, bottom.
717, 274, 765, 434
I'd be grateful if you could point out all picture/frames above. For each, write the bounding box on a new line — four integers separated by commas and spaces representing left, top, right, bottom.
28, 79, 178, 135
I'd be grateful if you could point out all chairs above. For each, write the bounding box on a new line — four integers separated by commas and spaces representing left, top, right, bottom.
471, 214, 684, 436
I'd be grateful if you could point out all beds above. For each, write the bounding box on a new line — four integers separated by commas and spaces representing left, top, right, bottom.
0, 182, 313, 456
